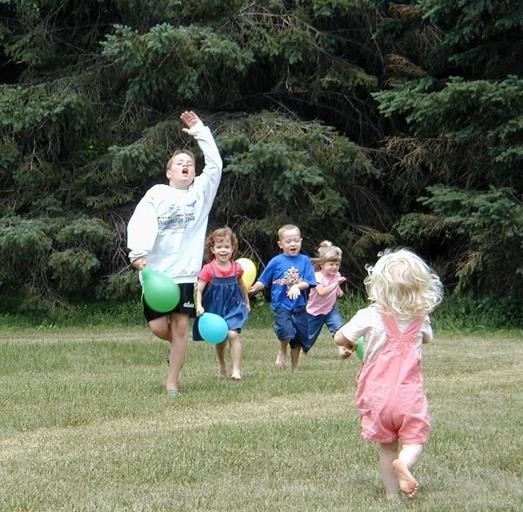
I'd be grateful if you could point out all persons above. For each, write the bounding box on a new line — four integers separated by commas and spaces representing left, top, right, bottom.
247, 222, 316, 370
300, 241, 352, 360
193, 227, 250, 381
334, 250, 446, 499
127, 109, 223, 397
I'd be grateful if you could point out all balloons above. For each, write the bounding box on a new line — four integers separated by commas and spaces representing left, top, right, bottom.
353, 336, 366, 364
197, 312, 228, 344
233, 257, 257, 290
140, 267, 181, 313
